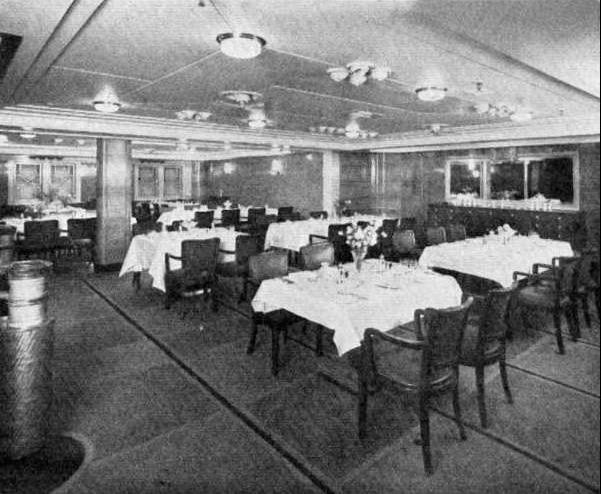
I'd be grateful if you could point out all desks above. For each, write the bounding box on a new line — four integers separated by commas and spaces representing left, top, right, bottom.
117, 226, 250, 298
418, 224, 575, 288
263, 212, 399, 252
426, 204, 587, 250
250, 257, 465, 392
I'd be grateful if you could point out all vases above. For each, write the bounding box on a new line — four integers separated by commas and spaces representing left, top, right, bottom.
335, 217, 381, 274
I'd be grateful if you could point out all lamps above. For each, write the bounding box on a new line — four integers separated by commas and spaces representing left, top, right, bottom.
216, 32, 265, 59
94, 101, 121, 113
415, 86, 447, 103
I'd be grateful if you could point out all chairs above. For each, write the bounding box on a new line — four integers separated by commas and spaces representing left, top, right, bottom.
1, 199, 417, 311
246, 225, 601, 476
